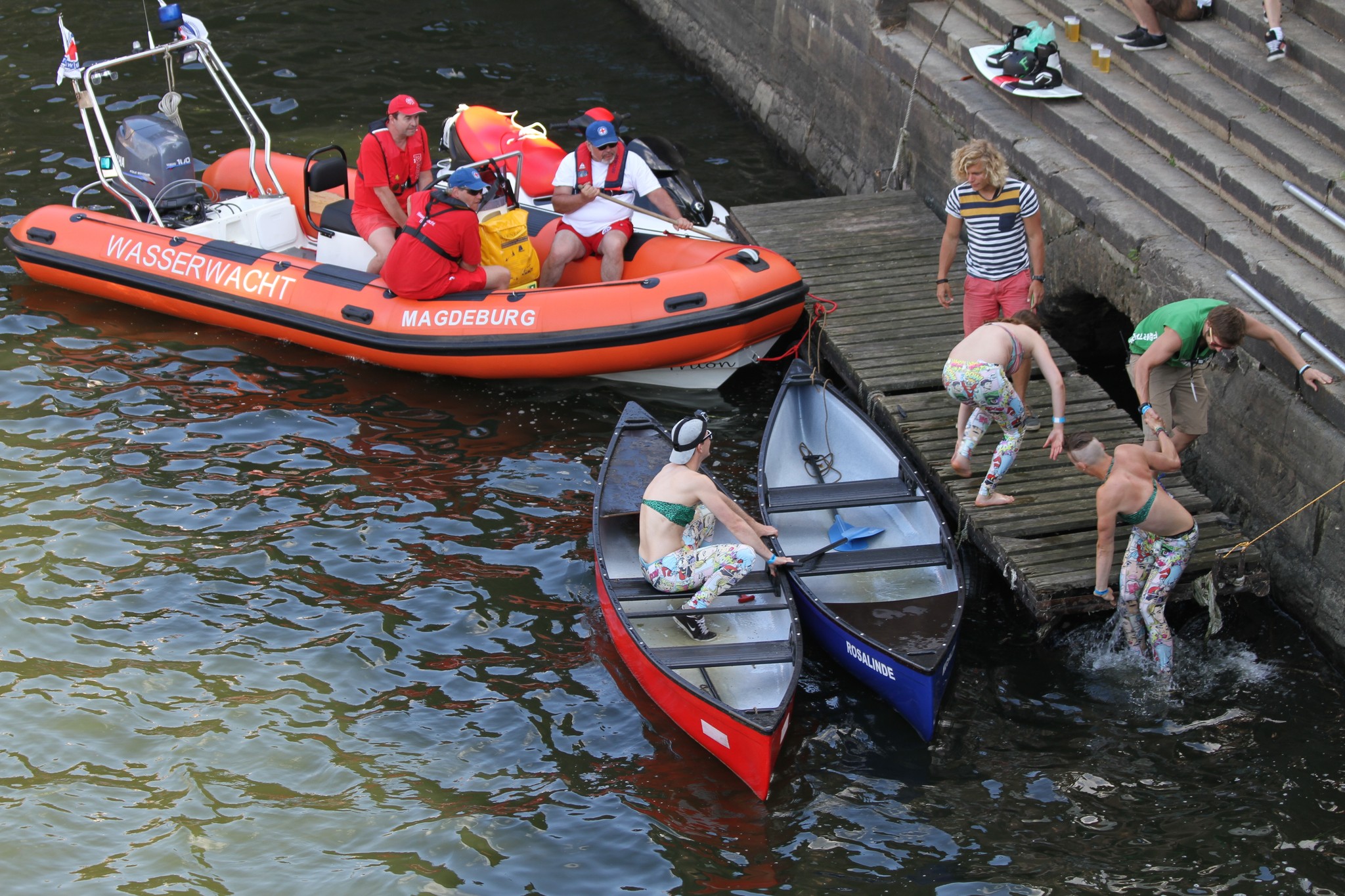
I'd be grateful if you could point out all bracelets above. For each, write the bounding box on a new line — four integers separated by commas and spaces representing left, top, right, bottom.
1298, 364, 1310, 378
1155, 426, 1168, 436
1140, 404, 1150, 414
1093, 589, 1108, 596
765, 553, 776, 564
1052, 416, 1066, 424
936, 278, 948, 284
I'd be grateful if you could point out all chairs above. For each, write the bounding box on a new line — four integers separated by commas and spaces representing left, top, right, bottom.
302, 144, 379, 274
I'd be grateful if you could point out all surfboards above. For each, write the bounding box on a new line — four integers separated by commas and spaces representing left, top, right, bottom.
968, 26, 1083, 101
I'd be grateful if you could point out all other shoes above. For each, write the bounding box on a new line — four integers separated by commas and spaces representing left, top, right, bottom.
1017, 40, 1064, 89
985, 25, 1032, 69
1022, 405, 1041, 430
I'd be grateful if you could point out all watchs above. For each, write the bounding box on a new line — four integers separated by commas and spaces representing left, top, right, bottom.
1032, 274, 1046, 282
1137, 402, 1152, 414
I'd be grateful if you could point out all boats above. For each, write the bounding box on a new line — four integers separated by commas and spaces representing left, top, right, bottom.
590, 400, 804, 800
3, 0, 812, 391
755, 357, 968, 747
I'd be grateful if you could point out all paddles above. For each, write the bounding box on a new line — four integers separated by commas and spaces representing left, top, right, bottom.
778, 520, 881, 578
574, 181, 729, 245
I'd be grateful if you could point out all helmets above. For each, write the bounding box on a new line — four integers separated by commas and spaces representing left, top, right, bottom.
1003, 51, 1038, 78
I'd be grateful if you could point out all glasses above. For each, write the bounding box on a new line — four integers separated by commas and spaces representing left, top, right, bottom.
598, 142, 618, 151
699, 430, 713, 444
1208, 326, 1235, 350
458, 187, 483, 195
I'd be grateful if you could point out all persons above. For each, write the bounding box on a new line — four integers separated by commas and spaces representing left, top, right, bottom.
377, 166, 512, 302
1124, 298, 1333, 499
1263, 0, 1287, 63
350, 94, 429, 277
941, 309, 1066, 507
1113, 0, 1212, 50
638, 414, 793, 643
1062, 418, 1199, 673
936, 138, 1045, 339
535, 120, 694, 288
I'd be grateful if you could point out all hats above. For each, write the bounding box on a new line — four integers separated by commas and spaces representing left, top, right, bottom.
669, 417, 706, 465
448, 167, 491, 191
387, 94, 428, 116
585, 120, 618, 147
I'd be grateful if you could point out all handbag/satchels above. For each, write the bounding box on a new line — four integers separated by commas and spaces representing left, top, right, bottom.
477, 208, 541, 285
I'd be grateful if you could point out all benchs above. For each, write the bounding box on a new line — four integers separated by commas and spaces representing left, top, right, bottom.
648, 640, 799, 669
607, 569, 778, 600
788, 526, 953, 577
762, 461, 919, 509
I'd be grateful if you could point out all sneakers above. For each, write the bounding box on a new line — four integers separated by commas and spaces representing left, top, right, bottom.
1264, 30, 1286, 63
1123, 33, 1168, 52
672, 605, 717, 641
1261, 1, 1283, 23
1114, 25, 1147, 42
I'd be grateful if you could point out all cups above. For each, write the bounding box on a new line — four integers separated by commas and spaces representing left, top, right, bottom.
1064, 16, 1076, 39
1091, 44, 1103, 67
1099, 49, 1110, 73
1068, 19, 1080, 42
